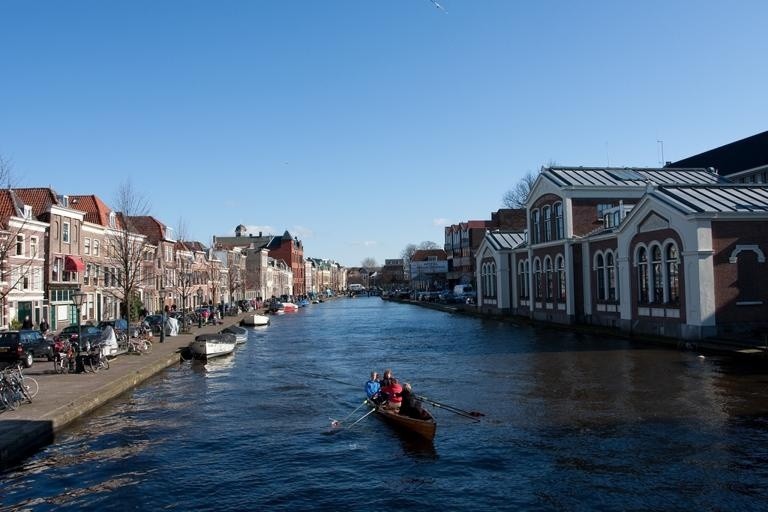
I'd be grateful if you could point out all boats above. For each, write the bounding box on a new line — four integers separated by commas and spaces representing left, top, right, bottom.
364, 390, 437, 446
221, 324, 250, 344
191, 333, 238, 362
242, 310, 271, 326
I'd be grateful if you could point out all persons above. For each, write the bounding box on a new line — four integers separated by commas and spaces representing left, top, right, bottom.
39, 318, 50, 340
364, 371, 382, 406
381, 378, 403, 411
393, 382, 422, 418
22, 315, 34, 331
379, 369, 396, 388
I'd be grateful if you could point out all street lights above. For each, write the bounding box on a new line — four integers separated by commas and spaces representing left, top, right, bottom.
196, 287, 205, 329
69, 286, 90, 375
158, 286, 170, 344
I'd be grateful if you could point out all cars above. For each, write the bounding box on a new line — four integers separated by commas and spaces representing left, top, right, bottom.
96, 318, 142, 339
410, 284, 475, 302
53, 323, 104, 344
140, 313, 179, 337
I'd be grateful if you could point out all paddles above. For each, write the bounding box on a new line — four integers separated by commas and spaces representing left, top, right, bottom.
414, 395, 485, 418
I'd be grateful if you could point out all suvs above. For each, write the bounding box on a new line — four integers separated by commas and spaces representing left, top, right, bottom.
0, 329, 56, 369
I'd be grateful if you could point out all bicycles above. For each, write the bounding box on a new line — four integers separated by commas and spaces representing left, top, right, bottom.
1, 360, 40, 414
52, 333, 153, 375
